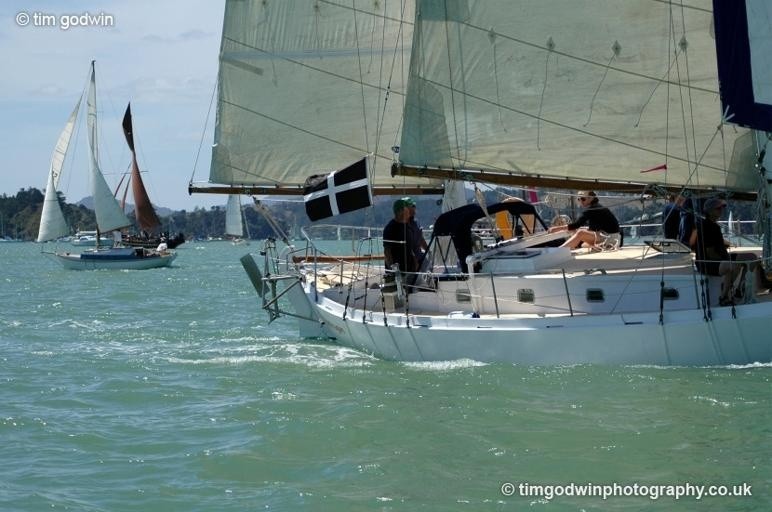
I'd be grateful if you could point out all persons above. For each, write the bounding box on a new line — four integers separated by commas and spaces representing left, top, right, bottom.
547, 189, 623, 252
112, 229, 122, 247
399, 197, 432, 272
691, 199, 757, 306
383, 200, 416, 294
146, 237, 168, 256
679, 197, 707, 252
662, 194, 690, 238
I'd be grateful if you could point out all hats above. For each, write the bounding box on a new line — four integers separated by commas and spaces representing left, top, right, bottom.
393, 197, 416, 215
704, 198, 728, 211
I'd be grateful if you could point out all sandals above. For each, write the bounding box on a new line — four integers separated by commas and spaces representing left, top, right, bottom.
718, 295, 737, 306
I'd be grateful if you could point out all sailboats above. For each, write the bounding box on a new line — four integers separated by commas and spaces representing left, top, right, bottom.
188, 0, 772, 367
37, 59, 186, 270
224, 194, 250, 245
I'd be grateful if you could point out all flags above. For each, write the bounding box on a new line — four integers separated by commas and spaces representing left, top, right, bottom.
304, 156, 374, 222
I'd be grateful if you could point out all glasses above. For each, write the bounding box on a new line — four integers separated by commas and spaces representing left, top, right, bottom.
576, 197, 587, 202
